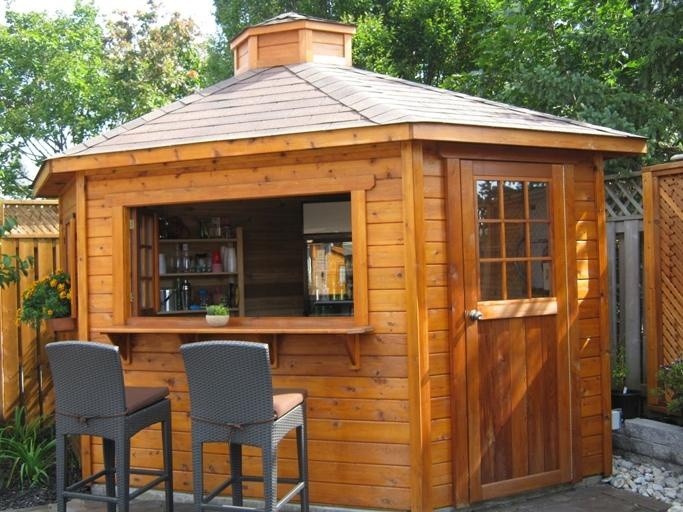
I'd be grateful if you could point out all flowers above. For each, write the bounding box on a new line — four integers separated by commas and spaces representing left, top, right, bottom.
17, 269, 72, 334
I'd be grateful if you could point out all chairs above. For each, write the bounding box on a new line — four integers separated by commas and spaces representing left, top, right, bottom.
44, 340, 173, 512
180, 340, 309, 511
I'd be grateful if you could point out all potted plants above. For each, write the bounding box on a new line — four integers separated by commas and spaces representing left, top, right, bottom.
610, 345, 642, 418
205, 302, 230, 328
648, 359, 683, 426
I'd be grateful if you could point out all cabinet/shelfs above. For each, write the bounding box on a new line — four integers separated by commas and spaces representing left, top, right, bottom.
156, 227, 245, 318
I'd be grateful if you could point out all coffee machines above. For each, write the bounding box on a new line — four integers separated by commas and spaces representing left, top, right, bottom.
298, 199, 352, 314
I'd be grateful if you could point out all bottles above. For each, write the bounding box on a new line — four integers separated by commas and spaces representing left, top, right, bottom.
176, 276, 236, 312
155, 242, 237, 274
198, 218, 234, 238
